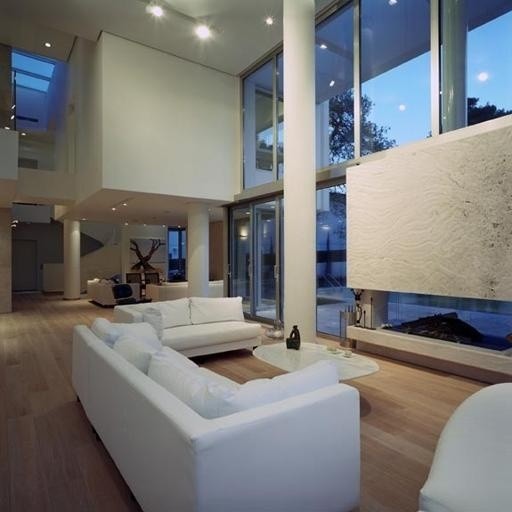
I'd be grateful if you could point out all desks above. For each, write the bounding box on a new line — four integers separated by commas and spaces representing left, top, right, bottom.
251, 342, 380, 418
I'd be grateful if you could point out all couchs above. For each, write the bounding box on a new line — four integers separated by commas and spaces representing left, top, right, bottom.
86, 278, 140, 308
74, 318, 359, 510
146, 277, 225, 302
114, 297, 262, 360
